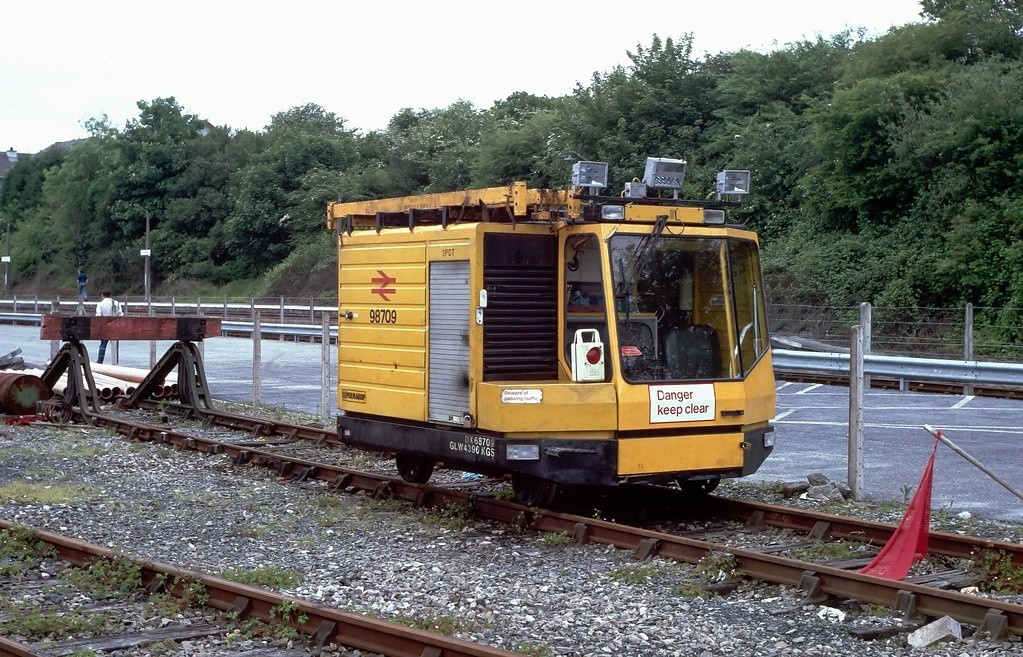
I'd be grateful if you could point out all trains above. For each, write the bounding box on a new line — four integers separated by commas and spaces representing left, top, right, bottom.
324, 159, 776, 513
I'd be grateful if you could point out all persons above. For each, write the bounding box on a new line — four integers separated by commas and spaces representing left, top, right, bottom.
94, 288, 124, 365
74, 269, 90, 302
